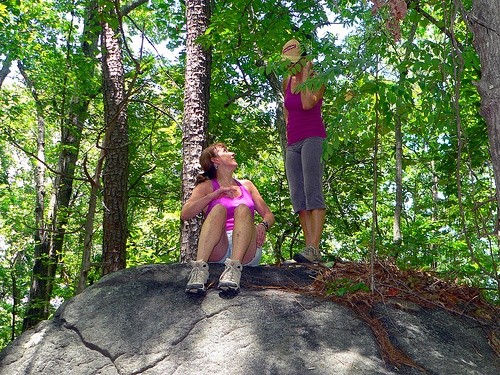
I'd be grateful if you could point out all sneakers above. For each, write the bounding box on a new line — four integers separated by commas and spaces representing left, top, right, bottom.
185, 260, 209, 293
218, 258, 242, 293
294, 246, 321, 263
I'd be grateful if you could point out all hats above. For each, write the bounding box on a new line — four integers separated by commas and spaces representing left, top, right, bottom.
281, 38, 307, 64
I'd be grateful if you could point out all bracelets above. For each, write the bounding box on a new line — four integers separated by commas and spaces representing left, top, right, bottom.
260, 221, 269, 230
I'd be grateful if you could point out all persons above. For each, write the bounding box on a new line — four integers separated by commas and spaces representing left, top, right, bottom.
279, 37, 326, 263
181, 143, 275, 293
